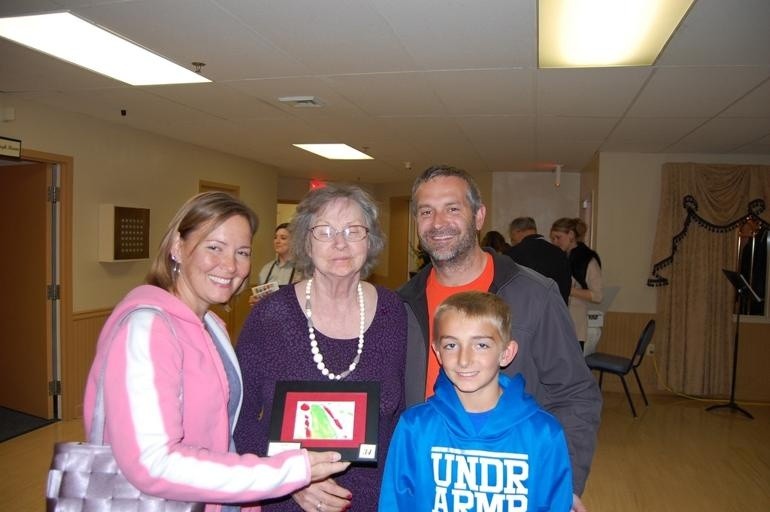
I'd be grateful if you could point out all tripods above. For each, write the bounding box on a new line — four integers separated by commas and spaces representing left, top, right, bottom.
705, 294, 754, 418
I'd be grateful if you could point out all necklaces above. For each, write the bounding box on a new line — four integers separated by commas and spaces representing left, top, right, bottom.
305, 279, 365, 381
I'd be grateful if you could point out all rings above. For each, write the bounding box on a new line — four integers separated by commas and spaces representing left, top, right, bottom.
316, 501, 322, 511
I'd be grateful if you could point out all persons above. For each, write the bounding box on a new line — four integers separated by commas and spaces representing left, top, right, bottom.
393, 166, 603, 512
480, 218, 604, 353
233, 184, 408, 512
249, 224, 313, 303
84, 191, 350, 512
376, 291, 574, 512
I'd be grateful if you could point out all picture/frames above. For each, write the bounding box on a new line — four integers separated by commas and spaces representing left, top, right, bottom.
266, 378, 379, 466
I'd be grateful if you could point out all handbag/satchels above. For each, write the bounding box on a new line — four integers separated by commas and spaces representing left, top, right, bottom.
46, 306, 205, 512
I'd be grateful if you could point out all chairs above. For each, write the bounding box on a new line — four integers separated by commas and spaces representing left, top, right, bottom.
585, 318, 657, 420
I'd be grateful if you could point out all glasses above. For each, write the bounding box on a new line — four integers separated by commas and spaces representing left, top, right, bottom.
306, 224, 369, 243
